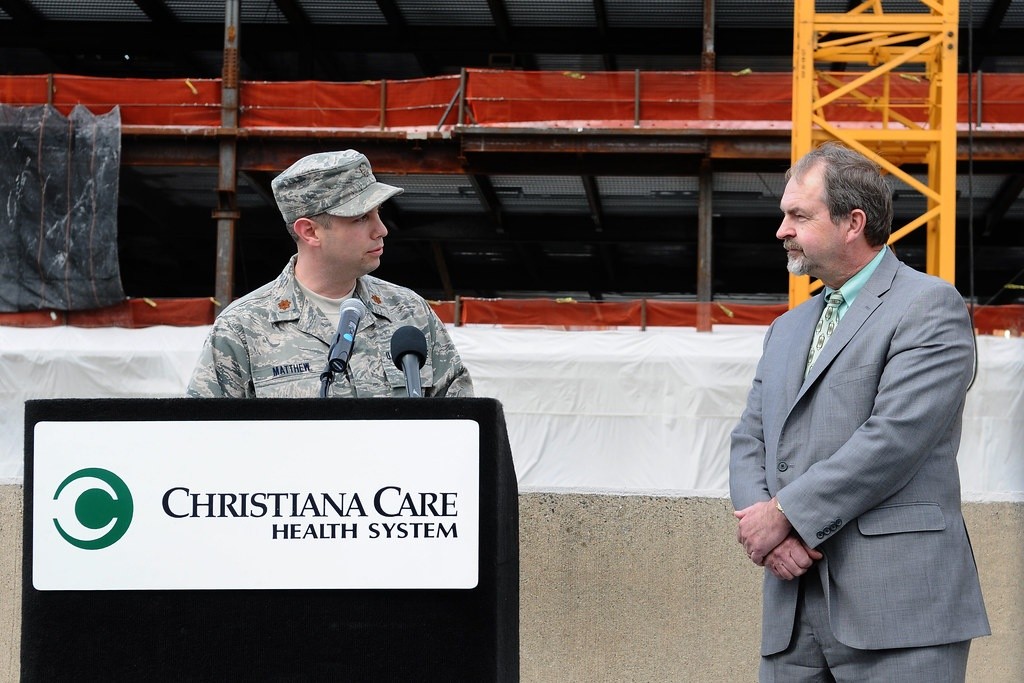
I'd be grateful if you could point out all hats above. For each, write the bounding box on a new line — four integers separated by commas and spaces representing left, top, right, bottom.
271, 149, 404, 224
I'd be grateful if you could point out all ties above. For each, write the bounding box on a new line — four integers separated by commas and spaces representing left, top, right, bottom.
800, 291, 845, 384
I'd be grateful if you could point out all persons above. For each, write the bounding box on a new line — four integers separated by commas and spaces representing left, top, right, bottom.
187, 150, 474, 398
729, 144, 991, 683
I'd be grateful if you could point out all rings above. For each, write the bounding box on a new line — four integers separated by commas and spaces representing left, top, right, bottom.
747, 552, 751, 557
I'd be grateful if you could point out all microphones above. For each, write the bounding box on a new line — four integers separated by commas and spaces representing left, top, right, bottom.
391, 326, 427, 397
329, 297, 367, 372
